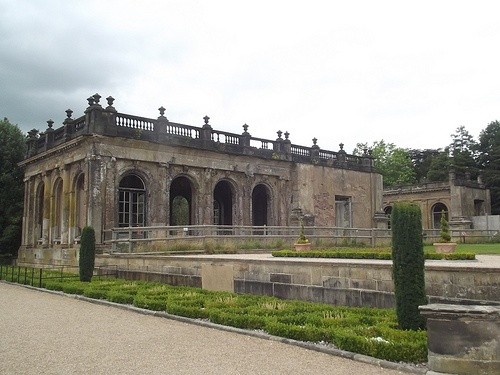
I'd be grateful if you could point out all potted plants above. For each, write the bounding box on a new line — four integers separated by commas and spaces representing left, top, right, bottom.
293, 219, 312, 252
432, 210, 458, 254
